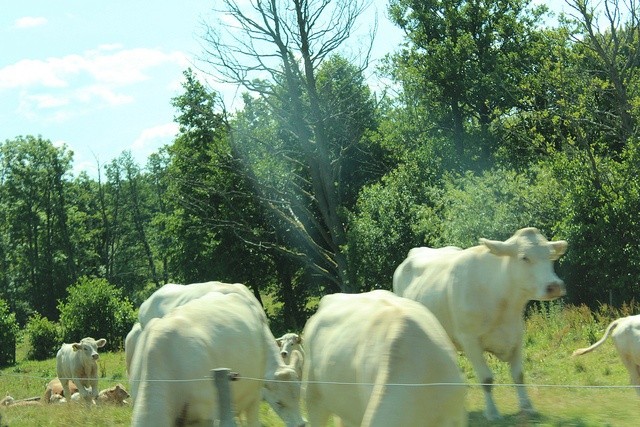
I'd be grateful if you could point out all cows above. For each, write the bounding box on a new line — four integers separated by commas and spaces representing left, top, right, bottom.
56, 338, 107, 401
98, 384, 130, 407
299, 289, 467, 427
392, 227, 568, 423
138, 281, 308, 427
59, 385, 97, 407
274, 333, 305, 379
571, 315, 639, 397
44, 377, 79, 403
125, 323, 142, 375
127, 292, 265, 427
